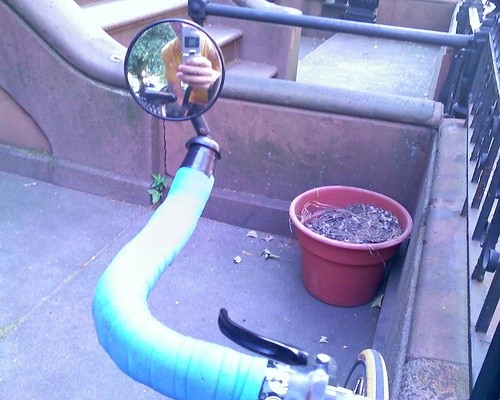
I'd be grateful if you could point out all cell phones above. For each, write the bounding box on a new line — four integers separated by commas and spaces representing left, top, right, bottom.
180, 27, 202, 71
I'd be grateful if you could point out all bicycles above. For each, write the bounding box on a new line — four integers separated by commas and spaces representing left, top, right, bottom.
91, 17, 391, 400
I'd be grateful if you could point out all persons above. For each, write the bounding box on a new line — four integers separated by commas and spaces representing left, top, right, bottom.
162, 21, 222, 118
